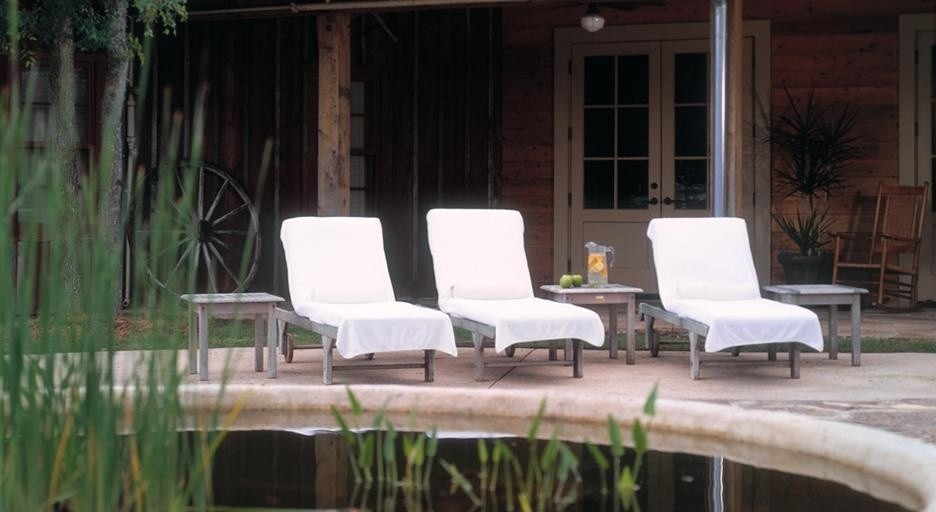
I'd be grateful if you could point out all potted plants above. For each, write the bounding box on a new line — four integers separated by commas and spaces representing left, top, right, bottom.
757, 85, 871, 284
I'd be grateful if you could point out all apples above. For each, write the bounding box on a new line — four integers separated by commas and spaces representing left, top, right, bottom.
559, 274, 572, 288
571, 274, 583, 287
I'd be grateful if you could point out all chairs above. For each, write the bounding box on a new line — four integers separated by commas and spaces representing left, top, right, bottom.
828, 181, 929, 313
276, 217, 457, 385
638, 217, 825, 380
426, 208, 606, 382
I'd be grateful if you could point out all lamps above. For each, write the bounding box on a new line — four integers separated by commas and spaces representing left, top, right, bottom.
580, 3, 605, 33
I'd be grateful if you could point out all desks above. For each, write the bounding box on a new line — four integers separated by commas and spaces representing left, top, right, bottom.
540, 283, 643, 366
760, 284, 869, 366
180, 292, 286, 381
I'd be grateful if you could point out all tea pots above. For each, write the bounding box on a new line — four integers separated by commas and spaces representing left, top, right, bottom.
584, 241, 616, 289
